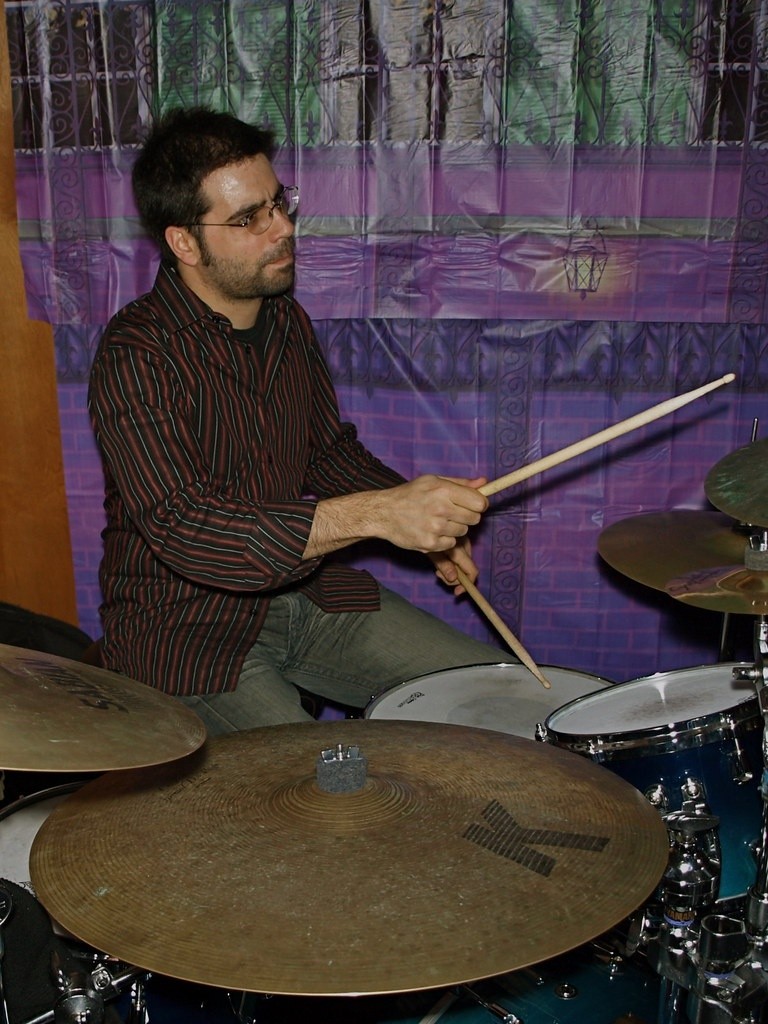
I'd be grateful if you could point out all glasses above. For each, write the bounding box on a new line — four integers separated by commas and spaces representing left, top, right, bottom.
175, 183, 300, 237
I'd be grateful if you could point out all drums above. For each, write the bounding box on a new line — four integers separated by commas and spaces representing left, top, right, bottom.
357, 663, 616, 741
535, 661, 768, 903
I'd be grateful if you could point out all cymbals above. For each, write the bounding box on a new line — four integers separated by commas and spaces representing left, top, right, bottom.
664, 566, 767, 615
596, 510, 768, 594
705, 437, 768, 528
0, 643, 207, 771
28, 719, 670, 996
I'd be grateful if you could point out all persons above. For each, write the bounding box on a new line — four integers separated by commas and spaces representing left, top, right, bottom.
85, 104, 528, 736
